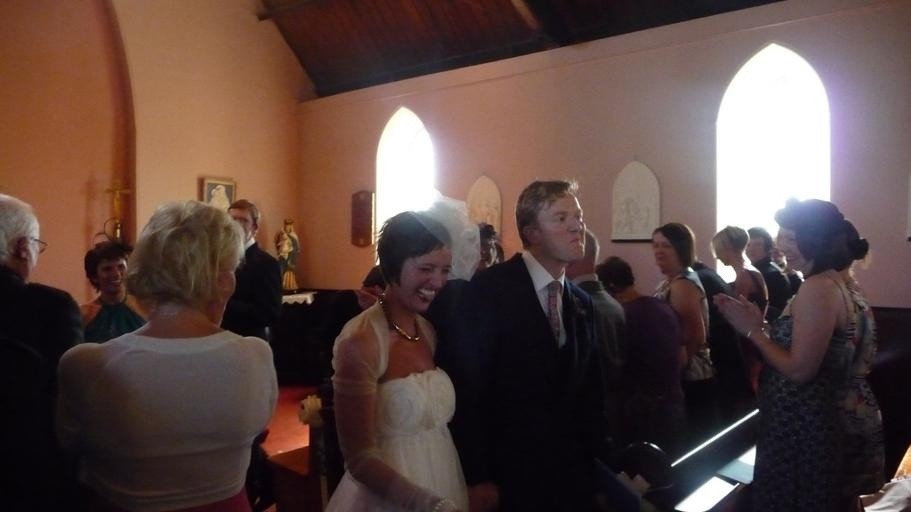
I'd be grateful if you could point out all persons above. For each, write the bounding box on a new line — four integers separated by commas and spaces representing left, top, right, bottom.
597, 255, 683, 435
56, 199, 279, 510
317, 215, 471, 512
0, 193, 86, 511
650, 224, 713, 416
743, 228, 789, 313
213, 199, 283, 512
689, 256, 738, 396
457, 223, 495, 277
74, 237, 143, 341
441, 178, 641, 509
561, 227, 626, 350
714, 200, 887, 511
711, 224, 769, 397
773, 239, 804, 293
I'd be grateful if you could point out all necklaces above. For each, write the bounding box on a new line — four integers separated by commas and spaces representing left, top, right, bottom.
378, 292, 424, 343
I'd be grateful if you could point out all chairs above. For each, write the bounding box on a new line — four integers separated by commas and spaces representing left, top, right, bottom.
268, 396, 334, 511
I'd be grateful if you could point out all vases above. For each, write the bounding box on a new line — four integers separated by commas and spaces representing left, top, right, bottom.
274, 219, 302, 294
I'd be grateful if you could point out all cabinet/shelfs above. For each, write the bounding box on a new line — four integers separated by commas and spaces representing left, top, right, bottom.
279, 289, 356, 386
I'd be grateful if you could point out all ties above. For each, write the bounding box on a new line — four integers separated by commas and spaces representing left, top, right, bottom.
547, 281, 568, 350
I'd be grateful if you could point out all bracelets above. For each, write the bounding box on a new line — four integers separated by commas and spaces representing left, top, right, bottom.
743, 320, 768, 338
429, 498, 456, 512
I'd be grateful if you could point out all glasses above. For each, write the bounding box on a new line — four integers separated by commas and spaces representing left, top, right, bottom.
28, 235, 49, 256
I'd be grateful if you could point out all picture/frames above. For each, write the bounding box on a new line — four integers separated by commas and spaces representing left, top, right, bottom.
201, 178, 236, 216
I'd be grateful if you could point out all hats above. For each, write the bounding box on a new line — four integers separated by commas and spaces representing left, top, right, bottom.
775, 198, 844, 233
